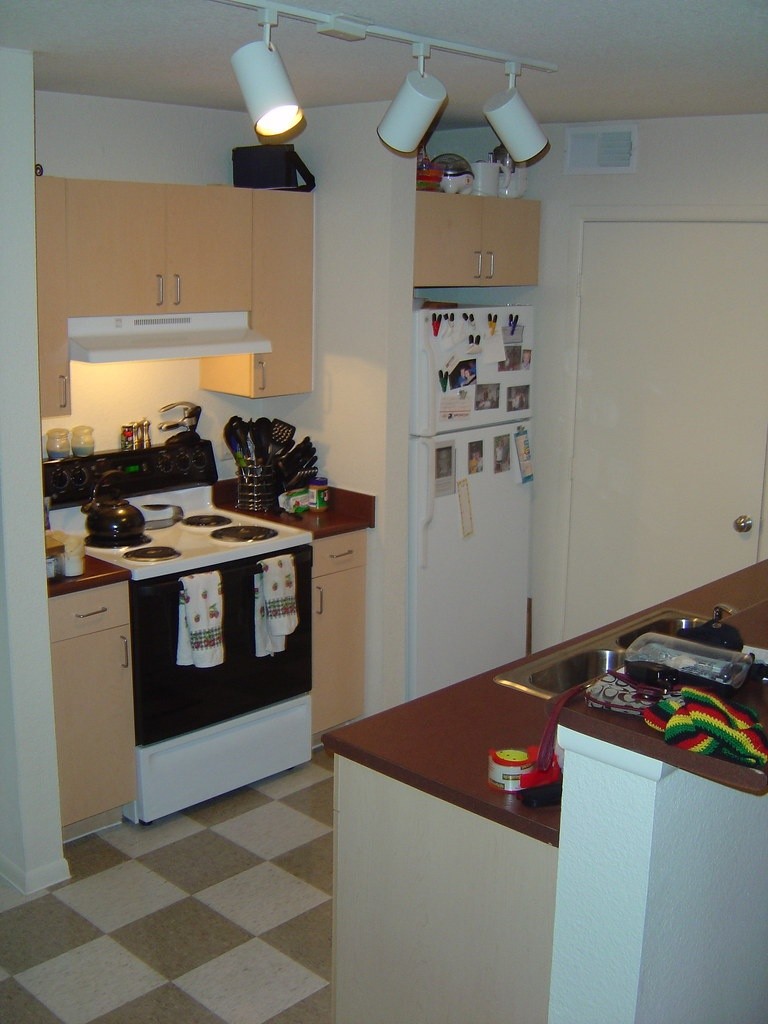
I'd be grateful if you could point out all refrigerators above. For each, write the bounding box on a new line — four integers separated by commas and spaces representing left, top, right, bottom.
406, 305, 532, 709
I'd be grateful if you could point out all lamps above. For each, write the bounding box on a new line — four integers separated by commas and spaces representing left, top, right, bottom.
378, 43, 446, 152
482, 60, 548, 163
230, 9, 303, 136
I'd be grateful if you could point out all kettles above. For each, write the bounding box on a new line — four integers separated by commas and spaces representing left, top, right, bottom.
81, 471, 144, 543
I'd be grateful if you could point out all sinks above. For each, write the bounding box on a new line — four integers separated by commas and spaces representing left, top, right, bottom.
588, 606, 713, 651
492, 641, 627, 701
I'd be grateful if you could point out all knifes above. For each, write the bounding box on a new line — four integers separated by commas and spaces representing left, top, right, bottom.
282, 435, 319, 493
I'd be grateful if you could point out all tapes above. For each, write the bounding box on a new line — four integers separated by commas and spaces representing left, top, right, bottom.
484, 741, 558, 789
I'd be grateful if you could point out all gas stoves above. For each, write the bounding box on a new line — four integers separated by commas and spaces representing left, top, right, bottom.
42, 439, 312, 581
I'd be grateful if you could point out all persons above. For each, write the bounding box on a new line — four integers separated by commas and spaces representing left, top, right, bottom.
522, 350, 530, 367
469, 451, 483, 473
496, 437, 510, 472
512, 391, 525, 410
477, 392, 490, 408
457, 367, 476, 385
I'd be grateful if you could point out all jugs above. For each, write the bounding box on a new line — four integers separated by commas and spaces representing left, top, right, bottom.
468, 161, 511, 198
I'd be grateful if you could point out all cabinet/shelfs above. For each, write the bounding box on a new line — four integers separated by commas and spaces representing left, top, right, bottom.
413, 191, 541, 286
35, 176, 314, 418
309, 527, 370, 749
47, 580, 138, 843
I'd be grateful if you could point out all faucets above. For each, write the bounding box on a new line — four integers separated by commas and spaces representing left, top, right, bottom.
713, 603, 734, 628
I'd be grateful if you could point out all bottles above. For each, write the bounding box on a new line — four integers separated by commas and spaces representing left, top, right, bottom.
46, 428, 70, 460
70, 425, 94, 457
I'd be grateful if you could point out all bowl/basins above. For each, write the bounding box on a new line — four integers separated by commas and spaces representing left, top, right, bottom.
441, 169, 473, 196
415, 161, 446, 192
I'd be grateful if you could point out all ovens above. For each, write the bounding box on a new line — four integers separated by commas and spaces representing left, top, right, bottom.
123, 545, 312, 750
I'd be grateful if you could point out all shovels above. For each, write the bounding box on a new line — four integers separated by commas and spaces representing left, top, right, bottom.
223, 413, 296, 511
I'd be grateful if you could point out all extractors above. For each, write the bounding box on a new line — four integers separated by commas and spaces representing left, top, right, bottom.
65, 311, 274, 367
